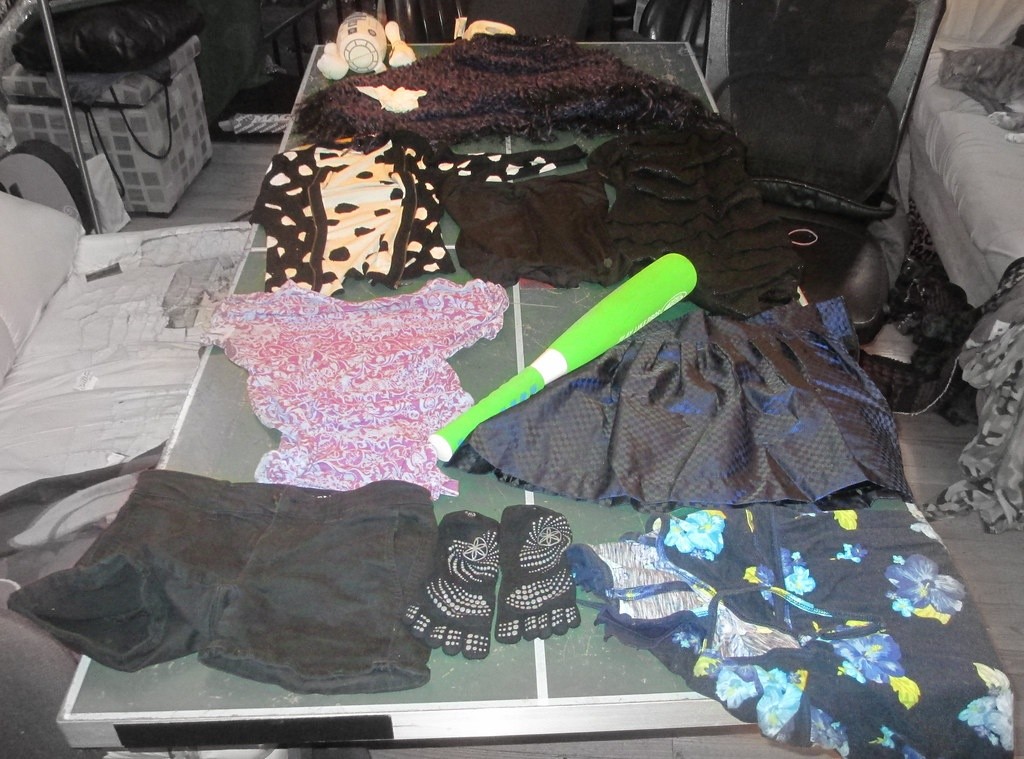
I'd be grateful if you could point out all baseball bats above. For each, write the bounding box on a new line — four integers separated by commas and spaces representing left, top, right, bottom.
429, 254, 697, 465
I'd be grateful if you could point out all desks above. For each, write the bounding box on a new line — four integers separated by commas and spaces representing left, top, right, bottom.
55, 42, 1015, 759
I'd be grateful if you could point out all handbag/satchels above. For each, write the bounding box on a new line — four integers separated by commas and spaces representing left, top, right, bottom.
11, 0, 204, 72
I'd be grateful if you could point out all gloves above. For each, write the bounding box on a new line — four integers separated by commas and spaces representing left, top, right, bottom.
402, 505, 581, 660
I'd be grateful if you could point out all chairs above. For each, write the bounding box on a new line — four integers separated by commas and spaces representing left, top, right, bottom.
703, 0, 946, 333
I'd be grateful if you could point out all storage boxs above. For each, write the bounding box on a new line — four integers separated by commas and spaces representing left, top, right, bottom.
3, 37, 213, 215
